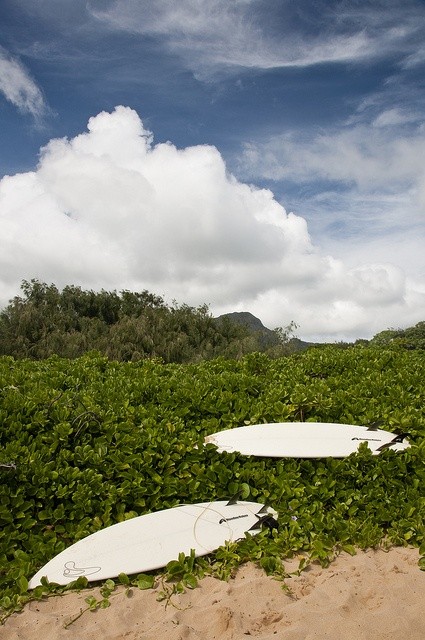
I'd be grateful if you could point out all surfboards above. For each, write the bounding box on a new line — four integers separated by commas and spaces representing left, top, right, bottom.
24, 488, 279, 590
204, 422, 410, 457
